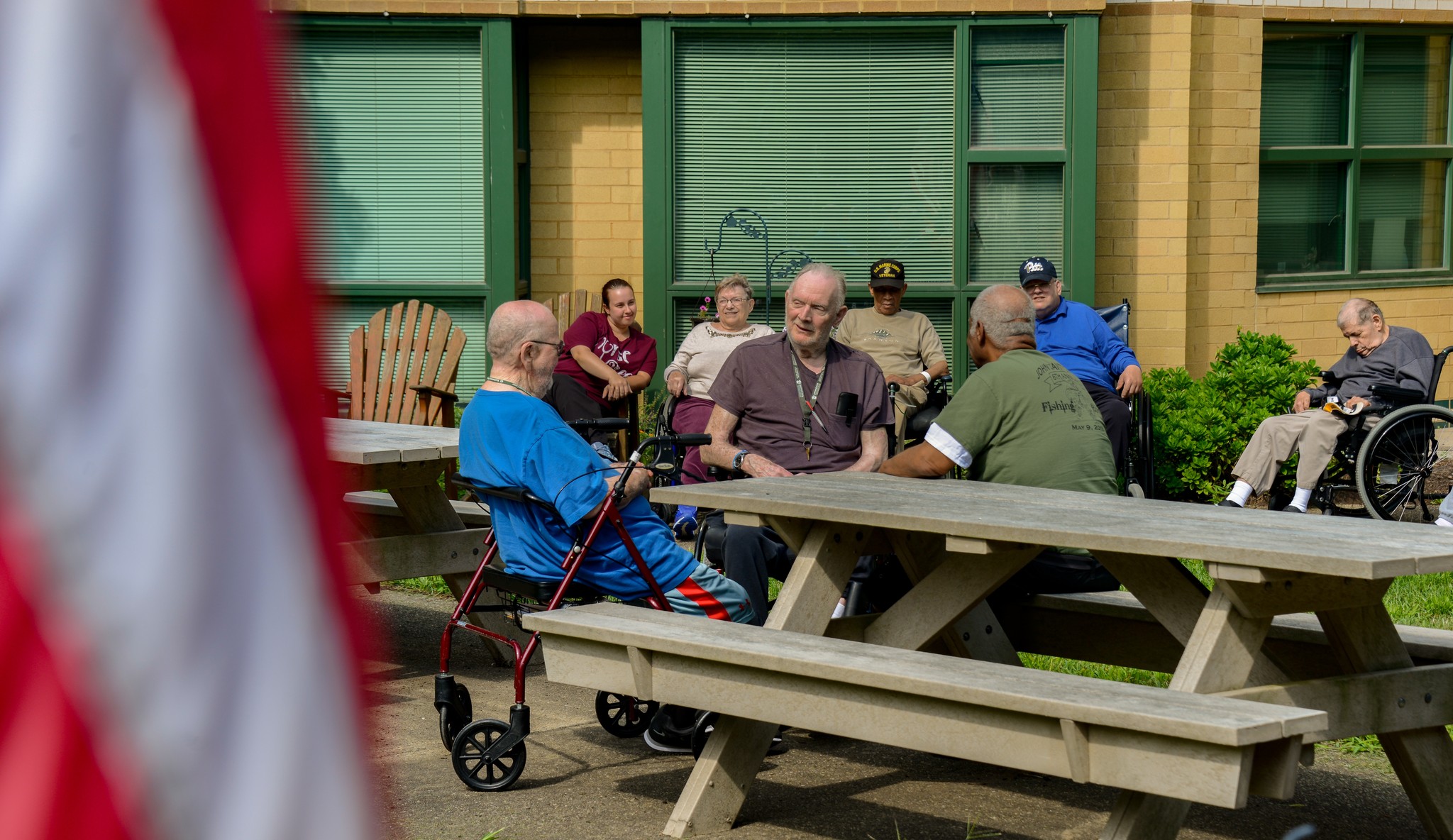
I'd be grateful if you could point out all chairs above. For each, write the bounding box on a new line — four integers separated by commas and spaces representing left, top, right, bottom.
543, 289, 639, 458
321, 300, 467, 497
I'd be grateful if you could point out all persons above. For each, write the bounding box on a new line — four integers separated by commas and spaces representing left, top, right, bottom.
835, 258, 950, 456
1215, 298, 1435, 514
873, 284, 1120, 597
665, 273, 776, 541
1018, 256, 1144, 478
457, 300, 783, 754
540, 279, 658, 463
699, 262, 895, 630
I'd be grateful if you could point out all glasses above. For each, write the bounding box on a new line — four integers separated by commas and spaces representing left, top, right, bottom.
531, 340, 565, 356
716, 298, 750, 305
1022, 280, 1057, 294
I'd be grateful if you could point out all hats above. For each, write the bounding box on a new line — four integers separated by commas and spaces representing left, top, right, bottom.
870, 259, 905, 289
1019, 257, 1058, 287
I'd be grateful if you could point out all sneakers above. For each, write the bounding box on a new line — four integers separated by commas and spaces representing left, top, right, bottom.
590, 442, 618, 462
644, 703, 693, 753
673, 513, 698, 540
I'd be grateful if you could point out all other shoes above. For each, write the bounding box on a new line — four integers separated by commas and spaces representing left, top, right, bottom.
1281, 505, 1307, 513
1214, 498, 1243, 508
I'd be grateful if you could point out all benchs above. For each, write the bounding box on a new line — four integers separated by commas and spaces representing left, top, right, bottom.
335, 489, 493, 596
991, 585, 1453, 680
523, 601, 1327, 809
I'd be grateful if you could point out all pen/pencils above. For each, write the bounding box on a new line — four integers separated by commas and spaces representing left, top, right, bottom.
806, 401, 829, 435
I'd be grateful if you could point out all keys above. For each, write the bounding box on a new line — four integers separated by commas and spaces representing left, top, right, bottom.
806, 448, 810, 460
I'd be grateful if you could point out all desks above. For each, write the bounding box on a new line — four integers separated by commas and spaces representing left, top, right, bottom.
320, 415, 530, 667
648, 474, 1453, 840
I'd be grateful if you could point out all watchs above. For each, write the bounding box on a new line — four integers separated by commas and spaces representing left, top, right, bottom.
732, 450, 750, 472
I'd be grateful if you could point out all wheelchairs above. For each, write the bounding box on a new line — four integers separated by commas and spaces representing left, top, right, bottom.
1258, 345, 1453, 522
886, 366, 966, 480
1093, 297, 1156, 499
693, 505, 904, 617
652, 375, 743, 525
434, 416, 741, 792
